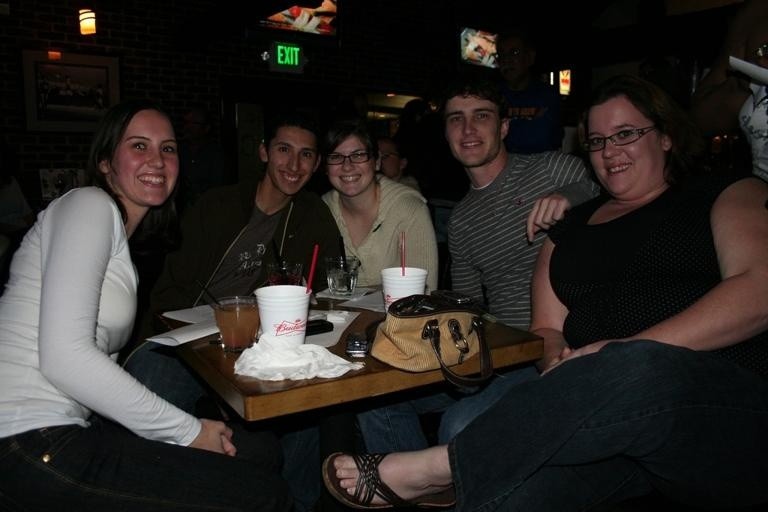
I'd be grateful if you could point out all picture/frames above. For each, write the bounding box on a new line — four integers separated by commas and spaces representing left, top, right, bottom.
23, 50, 121, 135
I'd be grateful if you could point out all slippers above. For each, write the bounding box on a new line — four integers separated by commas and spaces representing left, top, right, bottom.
321, 452, 456, 510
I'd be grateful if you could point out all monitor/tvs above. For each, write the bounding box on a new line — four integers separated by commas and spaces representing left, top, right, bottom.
459, 28, 500, 69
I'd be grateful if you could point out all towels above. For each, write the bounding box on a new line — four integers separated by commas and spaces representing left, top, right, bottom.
232, 329, 366, 379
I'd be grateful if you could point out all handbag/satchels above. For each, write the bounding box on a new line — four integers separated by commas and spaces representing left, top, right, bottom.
369, 295, 489, 373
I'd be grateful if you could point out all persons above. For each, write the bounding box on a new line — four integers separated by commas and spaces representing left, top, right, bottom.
318, 122, 438, 302
319, 70, 768, 509
355, 78, 604, 456
357, 33, 571, 211
738, 38, 767, 184
0, 152, 35, 263
1, 93, 321, 509
120, 114, 346, 423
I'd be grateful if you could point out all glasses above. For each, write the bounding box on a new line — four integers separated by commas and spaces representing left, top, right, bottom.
323, 152, 373, 165
582, 125, 657, 152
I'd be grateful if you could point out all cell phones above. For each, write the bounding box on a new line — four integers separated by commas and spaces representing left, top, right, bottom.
345, 329, 369, 355
306, 319, 333, 336
430, 290, 471, 304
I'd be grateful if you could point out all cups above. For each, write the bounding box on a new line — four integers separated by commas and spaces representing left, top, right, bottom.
210, 296, 263, 351
254, 284, 311, 346
380, 265, 428, 316
323, 254, 360, 297
